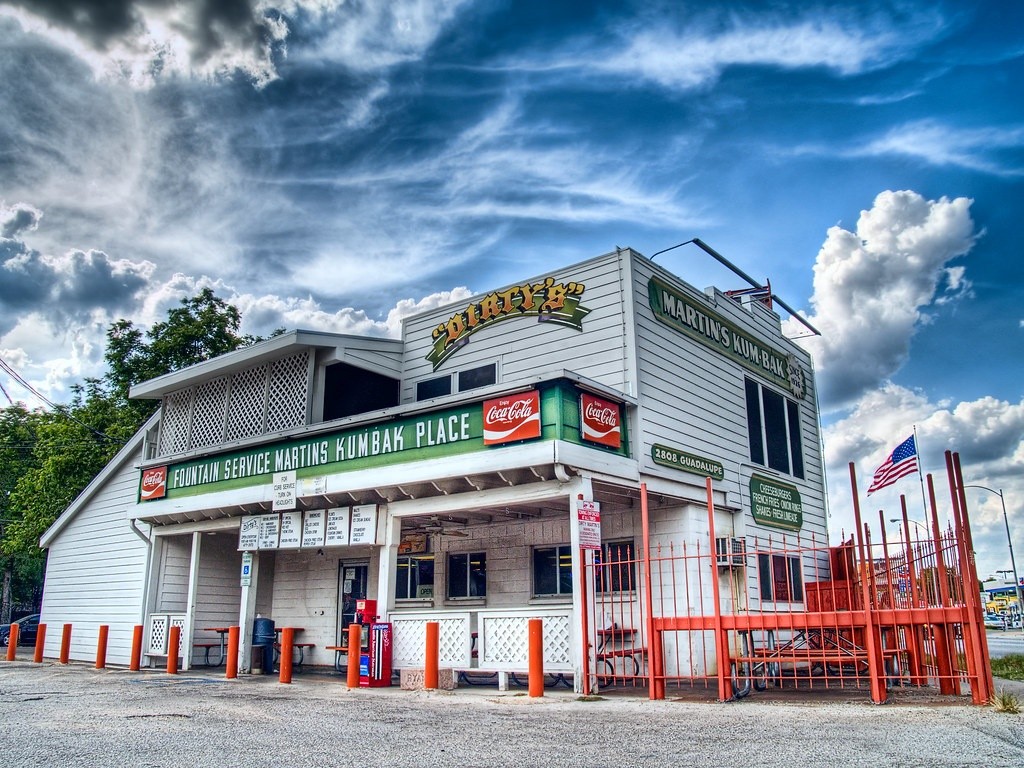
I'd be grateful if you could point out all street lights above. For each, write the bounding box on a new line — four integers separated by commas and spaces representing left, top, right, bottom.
954, 485, 1024, 629
890, 517, 939, 607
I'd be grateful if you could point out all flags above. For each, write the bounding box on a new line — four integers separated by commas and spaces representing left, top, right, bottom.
867, 432, 919, 497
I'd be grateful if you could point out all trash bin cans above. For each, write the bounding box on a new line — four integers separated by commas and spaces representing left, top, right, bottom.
251, 616, 276, 676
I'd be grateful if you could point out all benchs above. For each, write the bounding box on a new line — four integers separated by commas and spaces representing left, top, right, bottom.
729, 649, 907, 697
193, 643, 648, 691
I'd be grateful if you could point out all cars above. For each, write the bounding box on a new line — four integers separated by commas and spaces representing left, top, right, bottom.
0, 614, 40, 647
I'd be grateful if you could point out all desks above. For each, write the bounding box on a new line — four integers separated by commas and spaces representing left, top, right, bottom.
596, 629, 638, 650
204, 627, 305, 641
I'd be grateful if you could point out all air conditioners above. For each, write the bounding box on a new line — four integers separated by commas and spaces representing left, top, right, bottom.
714, 534, 747, 566
